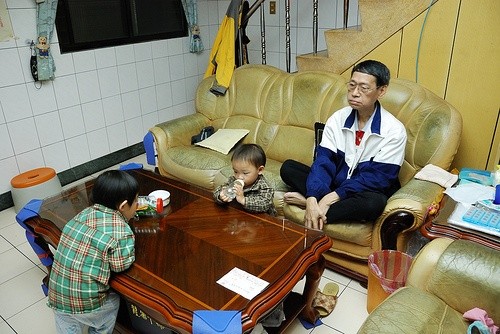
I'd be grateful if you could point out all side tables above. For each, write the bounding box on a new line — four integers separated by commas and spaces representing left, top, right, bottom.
421, 180, 500, 251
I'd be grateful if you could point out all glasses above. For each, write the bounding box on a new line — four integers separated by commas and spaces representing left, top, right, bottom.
345, 81, 380, 93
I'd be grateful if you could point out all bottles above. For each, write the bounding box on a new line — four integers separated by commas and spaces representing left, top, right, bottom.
138, 196, 163, 213
219, 179, 245, 199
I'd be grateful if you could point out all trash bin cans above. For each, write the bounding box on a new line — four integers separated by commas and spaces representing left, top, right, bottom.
367, 249, 413, 314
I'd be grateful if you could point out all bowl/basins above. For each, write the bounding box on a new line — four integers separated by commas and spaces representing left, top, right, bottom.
148, 190, 170, 207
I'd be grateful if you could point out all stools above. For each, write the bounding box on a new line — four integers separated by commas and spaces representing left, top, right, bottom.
11, 168, 62, 213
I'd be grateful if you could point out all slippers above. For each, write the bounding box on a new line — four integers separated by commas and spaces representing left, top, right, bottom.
312, 282, 339, 318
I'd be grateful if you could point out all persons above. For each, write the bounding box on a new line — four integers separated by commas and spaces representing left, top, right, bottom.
212, 144, 276, 215
46, 170, 140, 334
279, 60, 408, 231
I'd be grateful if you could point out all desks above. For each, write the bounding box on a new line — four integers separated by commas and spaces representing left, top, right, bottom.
15, 163, 333, 334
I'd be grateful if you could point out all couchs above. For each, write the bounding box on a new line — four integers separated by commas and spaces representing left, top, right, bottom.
148, 64, 463, 289
357, 238, 500, 334
